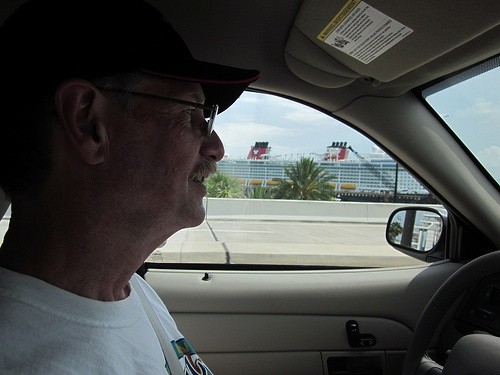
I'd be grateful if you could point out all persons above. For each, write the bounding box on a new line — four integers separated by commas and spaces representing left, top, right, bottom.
0, 0, 260, 375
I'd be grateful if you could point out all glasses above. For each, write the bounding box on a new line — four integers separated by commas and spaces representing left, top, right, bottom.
93, 83, 219, 137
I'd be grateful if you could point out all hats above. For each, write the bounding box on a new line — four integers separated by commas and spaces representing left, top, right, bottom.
0, 0, 262, 115
